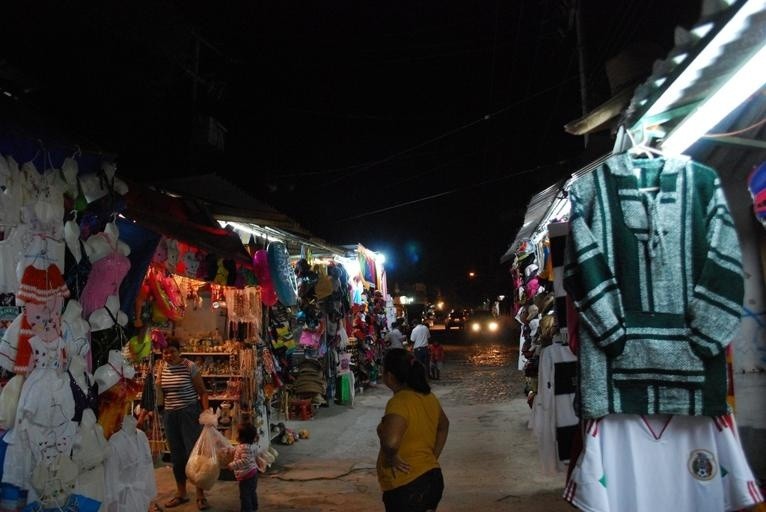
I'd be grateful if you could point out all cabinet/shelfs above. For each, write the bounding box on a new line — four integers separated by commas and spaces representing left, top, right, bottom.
125, 347, 272, 470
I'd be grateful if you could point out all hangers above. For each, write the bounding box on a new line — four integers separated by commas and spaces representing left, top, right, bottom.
29, 137, 57, 179
622, 123, 670, 196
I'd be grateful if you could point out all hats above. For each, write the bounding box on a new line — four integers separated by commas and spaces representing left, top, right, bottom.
509, 238, 539, 355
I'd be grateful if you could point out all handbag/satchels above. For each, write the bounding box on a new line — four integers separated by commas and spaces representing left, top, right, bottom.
153, 359, 164, 411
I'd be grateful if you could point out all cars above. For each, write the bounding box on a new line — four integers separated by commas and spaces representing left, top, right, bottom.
445, 312, 465, 331
466, 310, 501, 340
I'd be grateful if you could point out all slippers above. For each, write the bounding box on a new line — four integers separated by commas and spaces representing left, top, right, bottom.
163, 493, 189, 508
193, 497, 211, 511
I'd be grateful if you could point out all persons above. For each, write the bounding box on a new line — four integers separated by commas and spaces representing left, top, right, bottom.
58, 156, 156, 512
375, 347, 450, 512
218, 423, 259, 511
155, 336, 209, 511
383, 317, 444, 381
